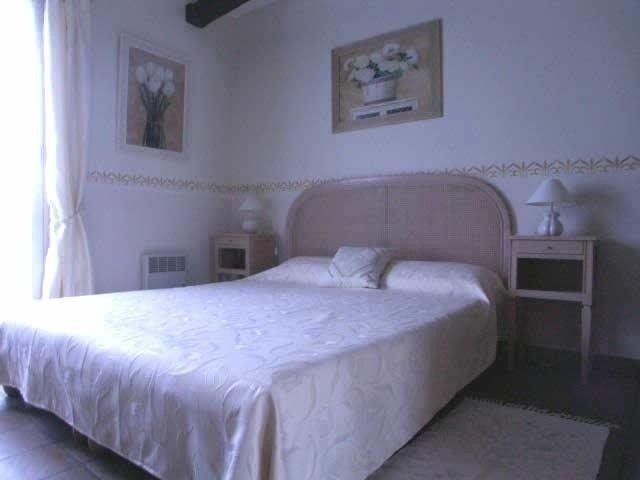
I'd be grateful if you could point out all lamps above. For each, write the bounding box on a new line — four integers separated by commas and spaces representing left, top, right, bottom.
526, 178, 578, 236
237, 198, 265, 235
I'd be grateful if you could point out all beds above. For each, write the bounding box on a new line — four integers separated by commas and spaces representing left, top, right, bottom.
0, 173, 514, 480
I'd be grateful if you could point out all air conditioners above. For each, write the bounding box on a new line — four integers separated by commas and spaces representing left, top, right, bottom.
142, 251, 190, 290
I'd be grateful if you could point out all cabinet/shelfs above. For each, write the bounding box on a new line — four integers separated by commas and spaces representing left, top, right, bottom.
351, 97, 418, 121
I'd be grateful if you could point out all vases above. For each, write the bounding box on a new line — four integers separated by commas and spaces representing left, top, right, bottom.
359, 74, 398, 106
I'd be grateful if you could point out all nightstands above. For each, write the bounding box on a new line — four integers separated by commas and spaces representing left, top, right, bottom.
212, 232, 278, 282
505, 235, 600, 387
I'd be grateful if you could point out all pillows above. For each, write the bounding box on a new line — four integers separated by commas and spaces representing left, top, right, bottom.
246, 246, 503, 305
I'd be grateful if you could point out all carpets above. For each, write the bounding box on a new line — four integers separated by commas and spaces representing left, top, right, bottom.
365, 394, 620, 480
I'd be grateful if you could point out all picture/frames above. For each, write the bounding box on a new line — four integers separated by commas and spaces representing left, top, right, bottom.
116, 32, 190, 159
331, 18, 443, 134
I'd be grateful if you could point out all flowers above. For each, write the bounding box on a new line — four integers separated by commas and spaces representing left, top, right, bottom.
135, 61, 176, 150
344, 43, 419, 87
